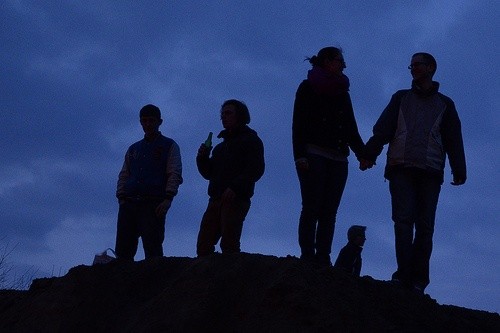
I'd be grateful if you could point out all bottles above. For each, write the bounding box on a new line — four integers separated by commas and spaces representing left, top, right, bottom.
204, 132, 213, 155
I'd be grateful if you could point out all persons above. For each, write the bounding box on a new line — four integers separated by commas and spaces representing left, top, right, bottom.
116, 104, 183, 260
292, 47, 366, 263
359, 53, 466, 292
334, 225, 367, 275
195, 100, 265, 253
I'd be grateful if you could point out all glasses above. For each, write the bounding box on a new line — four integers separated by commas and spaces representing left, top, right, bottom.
333, 59, 344, 65
408, 63, 429, 69
